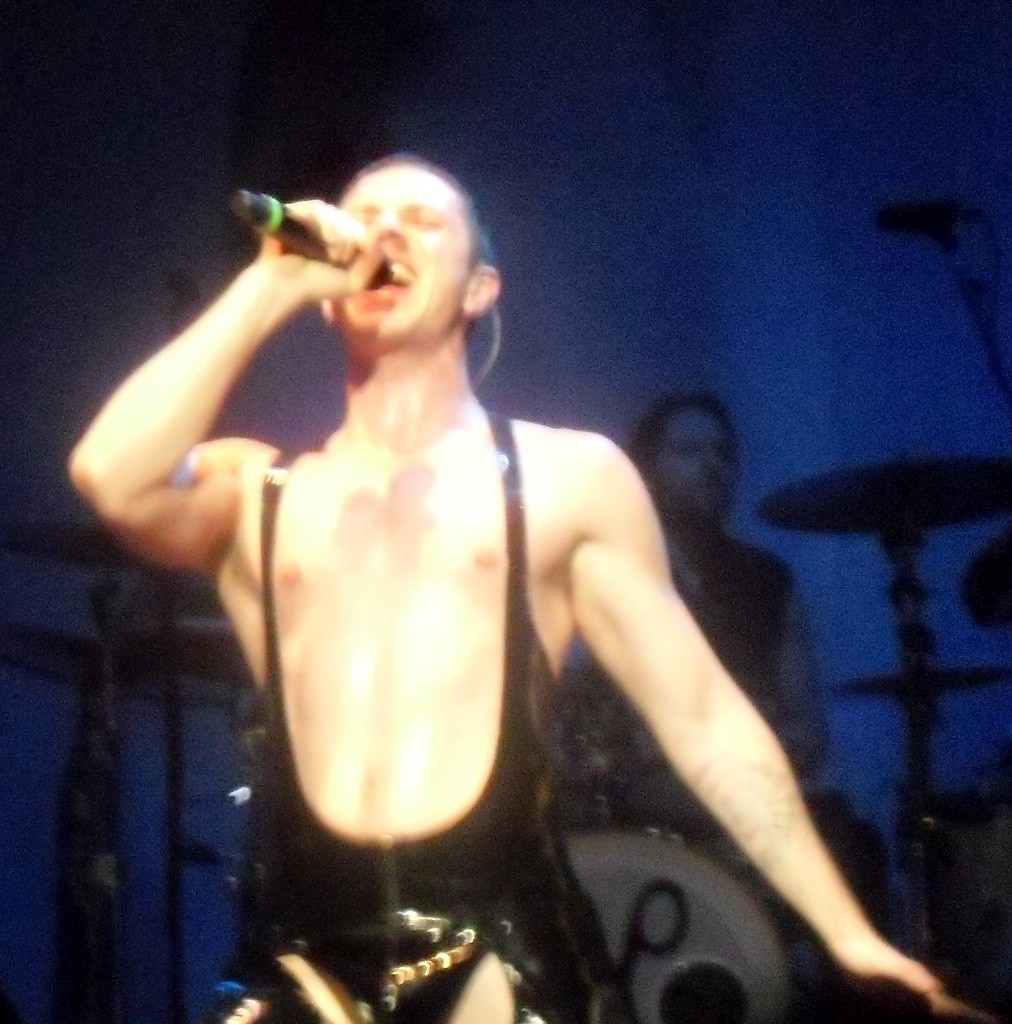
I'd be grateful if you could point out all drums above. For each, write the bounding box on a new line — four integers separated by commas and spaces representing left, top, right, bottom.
536, 790, 884, 1023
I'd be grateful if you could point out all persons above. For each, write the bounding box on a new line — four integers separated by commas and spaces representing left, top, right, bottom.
577, 384, 821, 849
69, 149, 1000, 1024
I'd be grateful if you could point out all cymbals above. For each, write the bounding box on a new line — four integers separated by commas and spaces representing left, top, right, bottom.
757, 455, 1010, 535
0, 523, 174, 578
0, 608, 243, 687
847, 659, 1007, 691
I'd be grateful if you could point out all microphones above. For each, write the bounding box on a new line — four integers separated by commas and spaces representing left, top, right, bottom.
230, 189, 392, 291
877, 197, 985, 235
